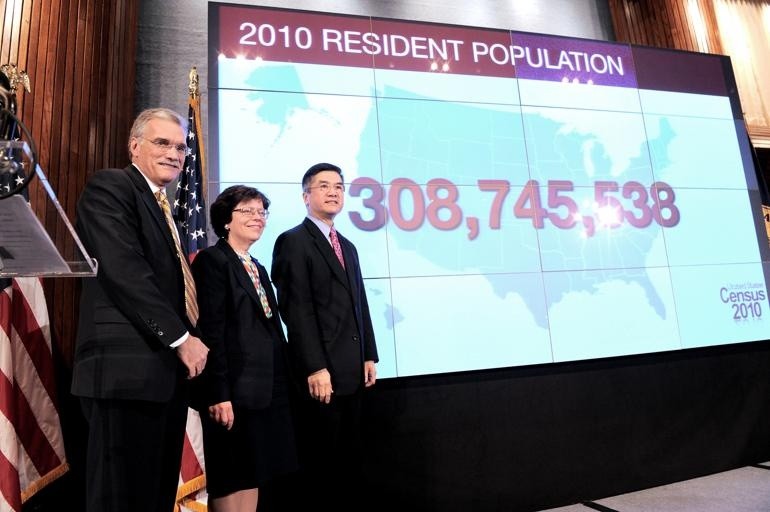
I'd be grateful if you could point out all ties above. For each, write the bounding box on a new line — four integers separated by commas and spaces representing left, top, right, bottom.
329, 225, 346, 271
155, 190, 199, 328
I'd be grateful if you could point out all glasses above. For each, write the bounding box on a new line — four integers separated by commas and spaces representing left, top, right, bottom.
135, 135, 189, 152
231, 205, 270, 219
304, 184, 344, 193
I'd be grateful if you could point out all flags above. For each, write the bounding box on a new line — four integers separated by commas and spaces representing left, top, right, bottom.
1, 100, 68, 512
174, 96, 208, 512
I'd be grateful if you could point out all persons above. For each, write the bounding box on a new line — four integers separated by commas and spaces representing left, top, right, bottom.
270, 163, 379, 512
70, 109, 207, 512
190, 186, 286, 512
